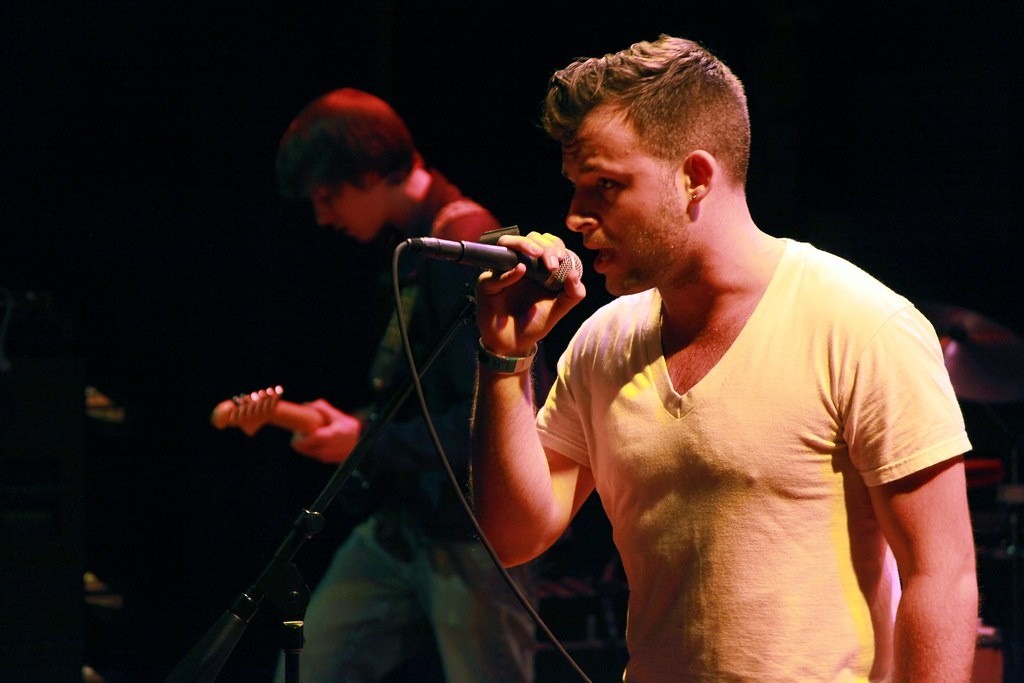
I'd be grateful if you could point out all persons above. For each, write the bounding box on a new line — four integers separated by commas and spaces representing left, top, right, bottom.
467, 31, 979, 682
291, 87, 536, 683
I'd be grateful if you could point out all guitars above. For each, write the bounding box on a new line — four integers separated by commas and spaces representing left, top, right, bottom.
211, 384, 328, 436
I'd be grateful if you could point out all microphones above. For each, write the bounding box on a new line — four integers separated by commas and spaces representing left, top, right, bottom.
404, 237, 583, 290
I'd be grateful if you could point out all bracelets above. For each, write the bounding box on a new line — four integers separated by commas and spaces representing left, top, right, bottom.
478, 337, 538, 373
